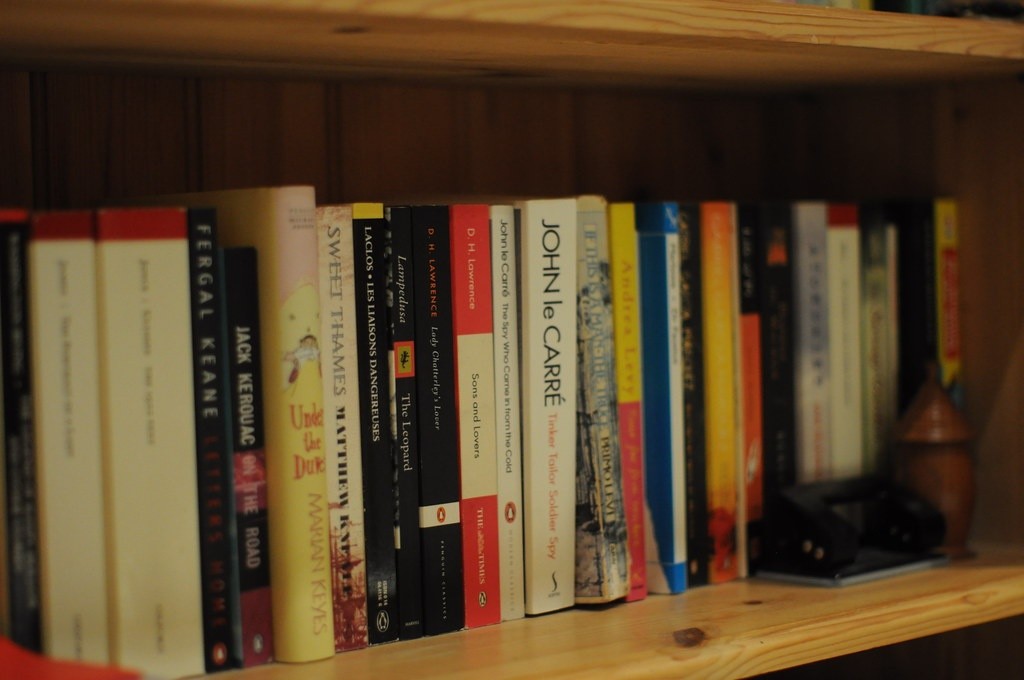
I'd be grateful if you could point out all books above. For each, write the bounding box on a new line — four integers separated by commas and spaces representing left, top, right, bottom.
0, 186, 963, 679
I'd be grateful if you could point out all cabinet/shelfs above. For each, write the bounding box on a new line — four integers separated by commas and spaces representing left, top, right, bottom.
0, 1, 1023, 680
